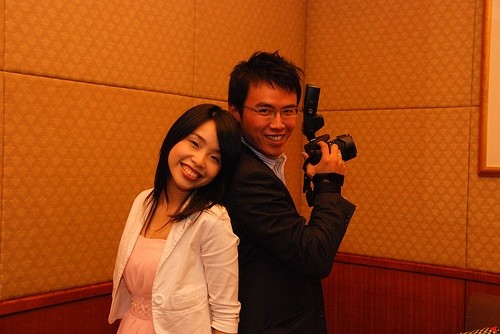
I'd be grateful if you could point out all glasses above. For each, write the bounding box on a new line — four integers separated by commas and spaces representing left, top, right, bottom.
243, 104, 299, 118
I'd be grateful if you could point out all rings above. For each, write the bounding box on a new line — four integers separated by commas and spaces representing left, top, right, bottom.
337, 154, 342, 156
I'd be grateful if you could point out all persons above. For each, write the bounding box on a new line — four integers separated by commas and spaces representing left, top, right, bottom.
209, 50, 358, 334
106, 104, 243, 334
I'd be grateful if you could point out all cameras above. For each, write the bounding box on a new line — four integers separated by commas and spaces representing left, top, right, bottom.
301, 84, 357, 166
